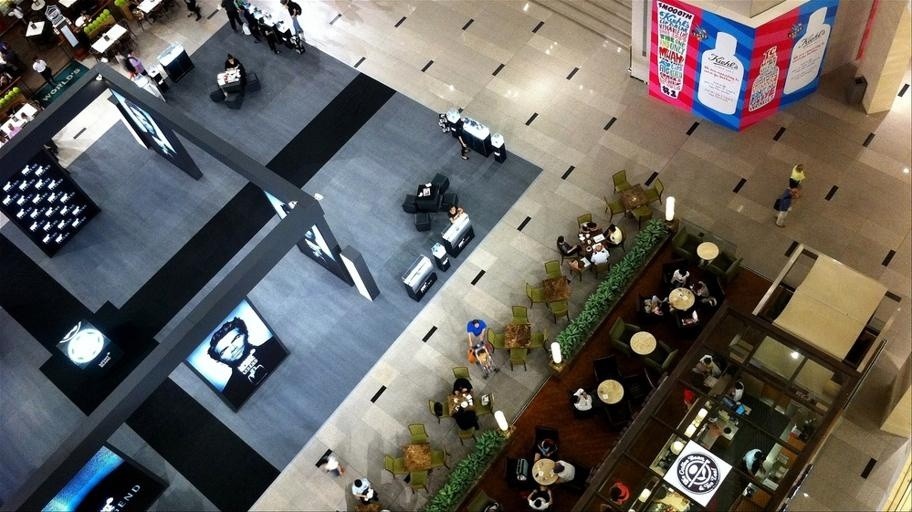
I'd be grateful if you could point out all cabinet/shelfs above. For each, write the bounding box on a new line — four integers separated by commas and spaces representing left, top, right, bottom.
630, 0, 839, 132
160, 45, 195, 83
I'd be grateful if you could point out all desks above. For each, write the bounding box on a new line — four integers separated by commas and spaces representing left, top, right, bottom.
728, 410, 818, 512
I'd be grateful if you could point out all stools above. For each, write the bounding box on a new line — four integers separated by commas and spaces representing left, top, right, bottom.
207, 82, 227, 103
244, 72, 262, 90
224, 91, 241, 107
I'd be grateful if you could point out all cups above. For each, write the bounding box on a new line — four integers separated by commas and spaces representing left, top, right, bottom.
578, 233, 592, 252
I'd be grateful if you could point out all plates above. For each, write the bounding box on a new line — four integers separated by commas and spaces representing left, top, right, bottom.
539, 472, 544, 476
682, 296, 689, 301
461, 401, 468, 408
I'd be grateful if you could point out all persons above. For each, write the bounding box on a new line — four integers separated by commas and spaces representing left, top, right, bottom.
207, 316, 288, 410
556, 235, 581, 257
451, 406, 479, 430
589, 245, 611, 265
112, 50, 138, 81
321, 455, 343, 476
688, 280, 718, 307
448, 205, 465, 224
605, 223, 623, 248
456, 115, 471, 160
526, 487, 555, 511
554, 460, 577, 484
789, 161, 806, 188
467, 319, 487, 346
696, 355, 722, 377
609, 480, 631, 506
184, 0, 201, 22
352, 477, 379, 505
578, 222, 600, 234
533, 439, 559, 463
127, 50, 149, 76
670, 266, 691, 288
680, 310, 700, 326
732, 380, 744, 402
569, 387, 598, 411
224, 54, 247, 75
8, 123, 21, 141
775, 187, 801, 228
451, 378, 472, 395
742, 448, 764, 480
32, 56, 58, 89
649, 294, 673, 316
21, 113, 58, 153
599, 503, 614, 511
220, 1, 304, 57
